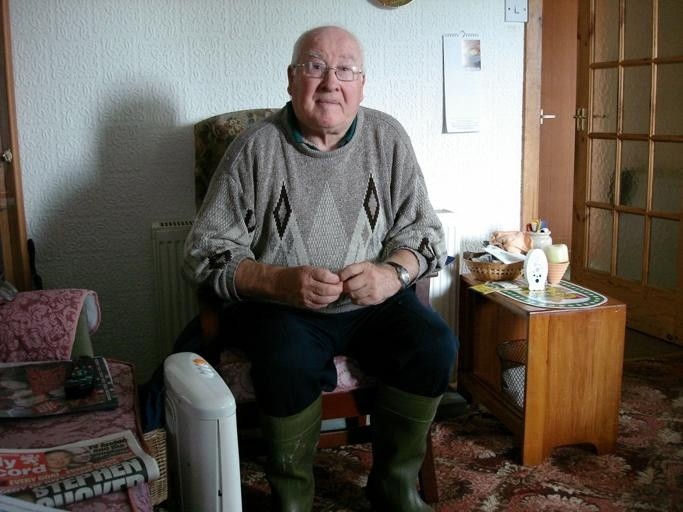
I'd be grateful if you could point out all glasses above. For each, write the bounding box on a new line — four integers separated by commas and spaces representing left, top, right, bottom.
295, 62, 362, 81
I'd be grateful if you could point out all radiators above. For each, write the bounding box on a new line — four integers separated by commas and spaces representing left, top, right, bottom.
149, 208, 461, 385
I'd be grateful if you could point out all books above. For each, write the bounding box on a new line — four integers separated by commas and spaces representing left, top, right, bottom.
0, 356, 119, 418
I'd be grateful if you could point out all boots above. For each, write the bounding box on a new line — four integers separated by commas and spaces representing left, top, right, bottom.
257, 395, 322, 511
367, 382, 445, 512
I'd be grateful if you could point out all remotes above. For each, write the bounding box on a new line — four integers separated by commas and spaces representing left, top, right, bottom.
64, 355, 96, 398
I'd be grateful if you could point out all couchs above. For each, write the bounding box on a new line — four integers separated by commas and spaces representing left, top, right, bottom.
0, 287, 159, 512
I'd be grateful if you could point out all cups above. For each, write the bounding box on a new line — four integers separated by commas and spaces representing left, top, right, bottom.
543, 244, 568, 263
525, 230, 552, 250
546, 261, 570, 284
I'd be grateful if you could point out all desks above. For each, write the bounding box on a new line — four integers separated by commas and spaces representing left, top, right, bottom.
457, 272, 629, 468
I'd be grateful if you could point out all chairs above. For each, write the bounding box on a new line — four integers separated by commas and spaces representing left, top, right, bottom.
178, 107, 442, 504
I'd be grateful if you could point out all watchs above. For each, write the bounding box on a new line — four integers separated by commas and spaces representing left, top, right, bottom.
385, 259, 410, 292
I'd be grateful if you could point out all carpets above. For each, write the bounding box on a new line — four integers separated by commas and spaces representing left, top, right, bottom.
245, 352, 683, 511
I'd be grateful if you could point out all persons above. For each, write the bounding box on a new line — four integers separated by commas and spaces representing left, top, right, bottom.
182, 26, 458, 511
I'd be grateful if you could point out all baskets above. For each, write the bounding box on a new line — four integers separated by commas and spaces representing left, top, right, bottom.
466, 261, 524, 282
144, 428, 169, 505
